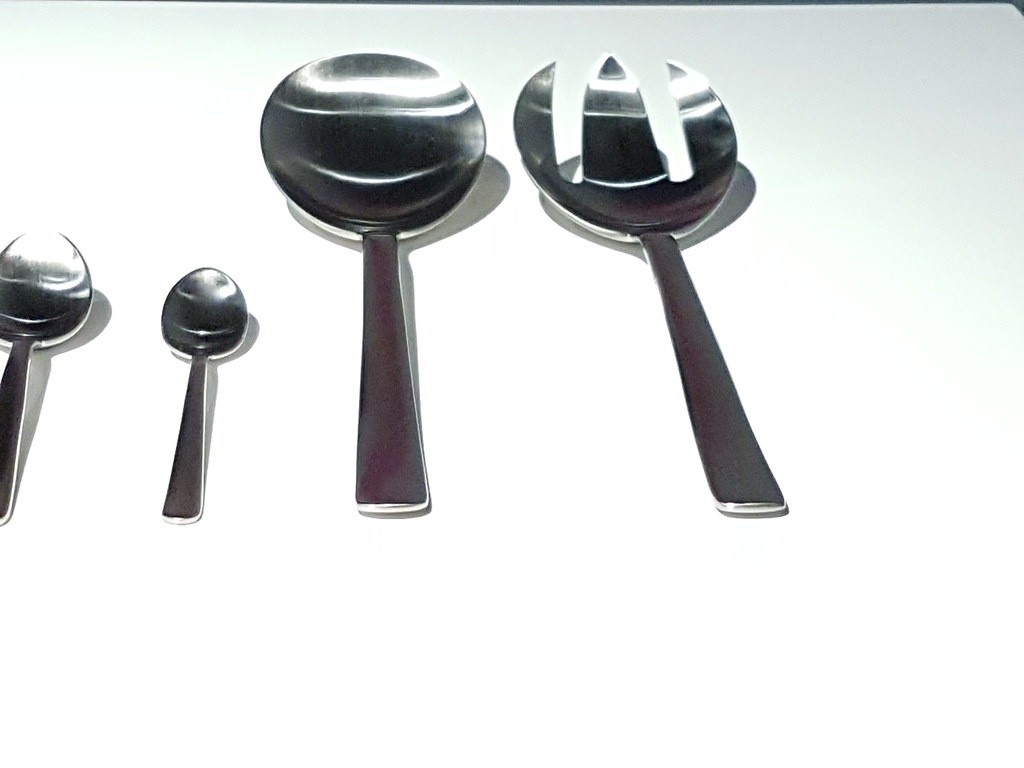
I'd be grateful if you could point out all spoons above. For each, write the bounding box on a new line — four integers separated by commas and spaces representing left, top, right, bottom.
261, 54, 489, 515
513, 54, 787, 515
0, 228, 94, 527
159, 267, 251, 525
